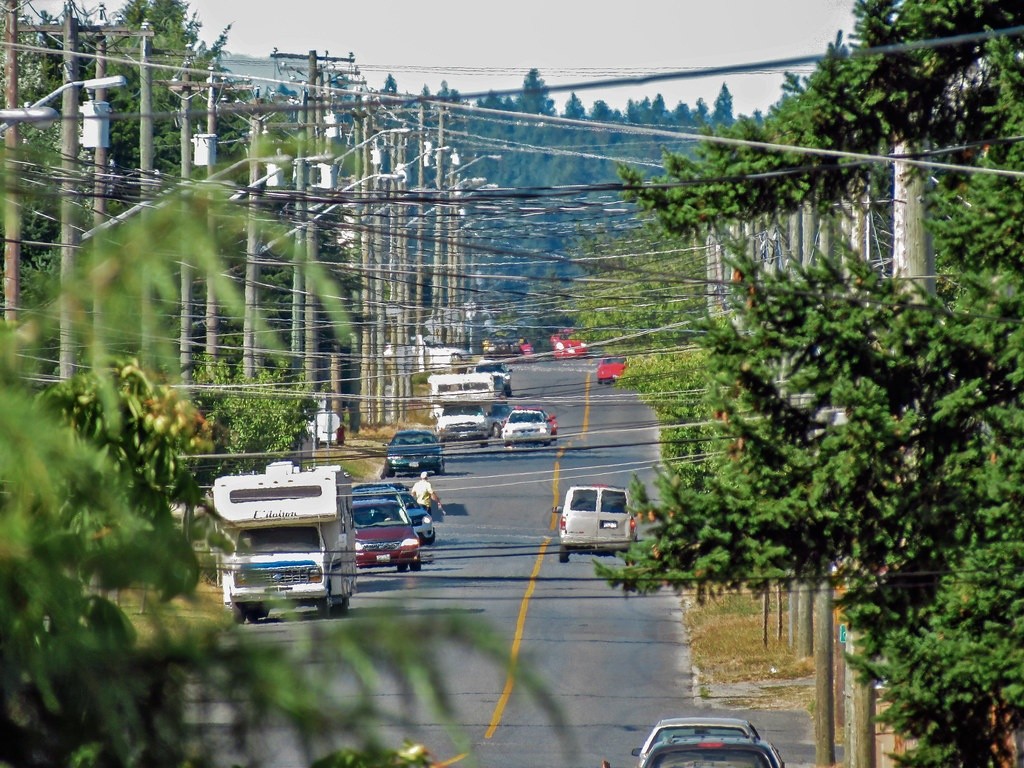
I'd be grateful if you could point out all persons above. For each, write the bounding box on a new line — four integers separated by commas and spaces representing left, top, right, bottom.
503, 369, 513, 397
411, 471, 442, 516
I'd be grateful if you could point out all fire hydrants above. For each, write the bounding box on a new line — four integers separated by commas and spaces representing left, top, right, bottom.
337, 426, 345, 444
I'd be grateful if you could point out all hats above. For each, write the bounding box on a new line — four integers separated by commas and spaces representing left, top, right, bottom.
506, 369, 512, 372
420, 472, 427, 478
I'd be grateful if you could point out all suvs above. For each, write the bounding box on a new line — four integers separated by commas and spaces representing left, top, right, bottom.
469, 359, 513, 397
399, 493, 435, 544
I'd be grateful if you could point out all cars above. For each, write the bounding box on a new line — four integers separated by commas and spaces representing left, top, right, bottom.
632, 716, 784, 768
500, 409, 552, 446
487, 404, 512, 437
502, 405, 558, 434
382, 430, 445, 477
482, 328, 534, 361
554, 329, 588, 360
596, 355, 629, 384
384, 339, 472, 366
351, 482, 422, 572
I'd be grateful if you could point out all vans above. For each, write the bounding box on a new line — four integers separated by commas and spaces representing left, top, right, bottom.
551, 485, 637, 566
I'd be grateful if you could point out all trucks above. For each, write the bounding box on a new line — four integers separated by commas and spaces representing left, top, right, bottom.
206, 461, 356, 624
428, 373, 495, 447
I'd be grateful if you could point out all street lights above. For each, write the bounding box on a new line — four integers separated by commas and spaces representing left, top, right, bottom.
61, 126, 503, 430
2, 74, 126, 132
